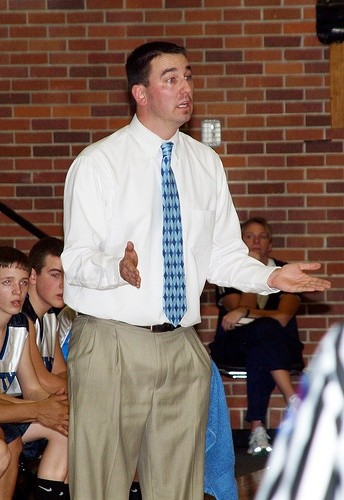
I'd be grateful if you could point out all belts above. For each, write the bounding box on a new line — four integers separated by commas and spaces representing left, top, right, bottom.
77, 312, 181, 332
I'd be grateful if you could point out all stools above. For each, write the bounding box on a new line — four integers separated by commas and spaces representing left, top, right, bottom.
219, 365, 301, 378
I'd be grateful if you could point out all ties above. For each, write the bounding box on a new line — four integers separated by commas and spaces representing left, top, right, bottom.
161, 142, 187, 327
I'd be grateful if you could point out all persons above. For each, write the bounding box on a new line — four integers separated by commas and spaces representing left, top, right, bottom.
254, 318, 343, 500
59, 41, 331, 500
0, 238, 143, 499
208, 217, 307, 455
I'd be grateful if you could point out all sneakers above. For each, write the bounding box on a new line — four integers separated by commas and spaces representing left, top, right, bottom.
246, 426, 272, 456
